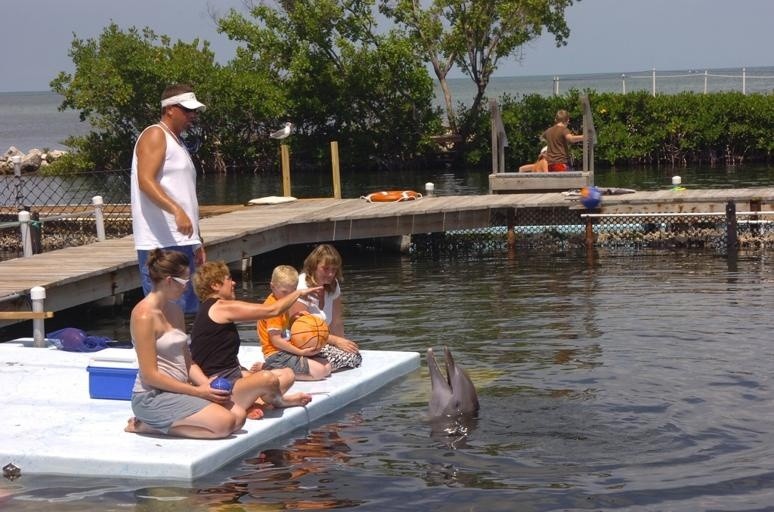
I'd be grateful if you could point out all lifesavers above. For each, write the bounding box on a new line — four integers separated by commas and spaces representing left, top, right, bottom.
367, 190, 418, 202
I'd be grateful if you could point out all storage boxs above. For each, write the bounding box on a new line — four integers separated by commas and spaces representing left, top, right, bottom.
87, 361, 137, 401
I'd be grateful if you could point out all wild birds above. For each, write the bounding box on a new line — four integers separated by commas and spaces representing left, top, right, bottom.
269, 121, 293, 145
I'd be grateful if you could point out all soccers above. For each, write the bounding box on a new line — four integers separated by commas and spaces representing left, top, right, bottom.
211, 377, 230, 390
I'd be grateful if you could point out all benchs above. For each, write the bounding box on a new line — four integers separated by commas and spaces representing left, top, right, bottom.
488, 172, 589, 194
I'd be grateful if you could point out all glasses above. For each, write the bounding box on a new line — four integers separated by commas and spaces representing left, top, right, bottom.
172, 277, 190, 288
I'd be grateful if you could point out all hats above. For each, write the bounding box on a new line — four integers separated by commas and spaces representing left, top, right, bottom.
161, 92, 206, 114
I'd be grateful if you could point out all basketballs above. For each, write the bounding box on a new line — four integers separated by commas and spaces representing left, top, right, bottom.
290, 315, 329, 351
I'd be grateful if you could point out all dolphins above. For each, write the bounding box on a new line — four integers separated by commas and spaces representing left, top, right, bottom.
427, 345, 480, 417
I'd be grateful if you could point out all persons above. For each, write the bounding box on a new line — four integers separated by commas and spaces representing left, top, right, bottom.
130, 84, 208, 336
296, 243, 361, 367
539, 109, 592, 171
519, 145, 548, 172
251, 264, 332, 380
125, 247, 248, 439
191, 259, 324, 420
